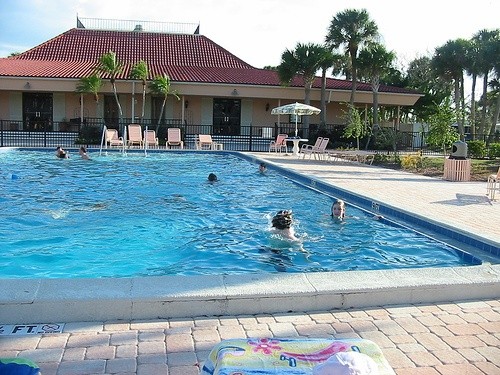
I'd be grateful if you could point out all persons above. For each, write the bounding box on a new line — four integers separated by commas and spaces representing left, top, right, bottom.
207, 174, 218, 184
56, 146, 71, 158
331, 199, 384, 221
79, 147, 89, 161
269, 208, 303, 245
259, 163, 267, 173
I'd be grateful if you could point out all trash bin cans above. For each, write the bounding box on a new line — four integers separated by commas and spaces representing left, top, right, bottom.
451, 141, 467, 181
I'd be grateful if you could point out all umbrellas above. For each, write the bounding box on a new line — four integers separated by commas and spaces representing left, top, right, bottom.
271, 101, 322, 137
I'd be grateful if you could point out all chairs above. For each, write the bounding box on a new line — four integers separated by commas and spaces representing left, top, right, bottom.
105, 129, 124, 150
487, 166, 500, 200
297, 137, 322, 161
268, 134, 288, 156
165, 128, 184, 149
201, 335, 397, 375
309, 138, 329, 163
143, 130, 159, 150
128, 124, 142, 149
195, 134, 217, 151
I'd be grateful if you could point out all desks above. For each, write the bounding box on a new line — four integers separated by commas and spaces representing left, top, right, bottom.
328, 150, 377, 167
284, 139, 309, 157
214, 143, 224, 150
